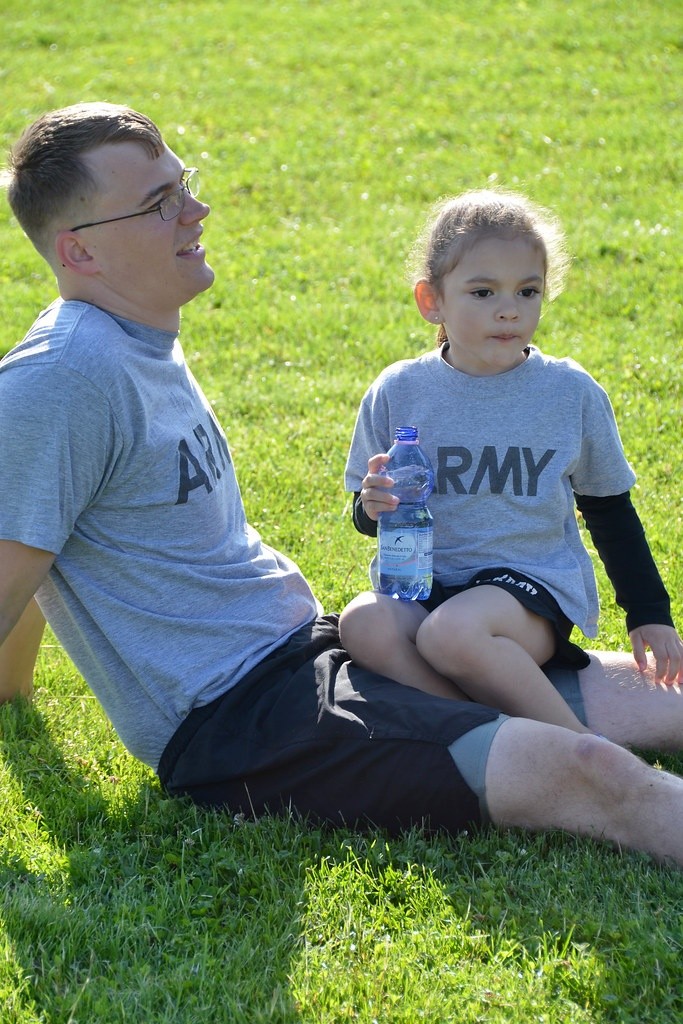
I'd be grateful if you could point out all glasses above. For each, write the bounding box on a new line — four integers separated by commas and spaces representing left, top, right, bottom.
69, 167, 200, 234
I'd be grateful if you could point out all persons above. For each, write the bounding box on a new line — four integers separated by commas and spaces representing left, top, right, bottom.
335, 184, 683, 741
1, 102, 683, 876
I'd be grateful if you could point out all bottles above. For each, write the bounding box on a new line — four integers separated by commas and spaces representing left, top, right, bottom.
377, 427, 433, 601
